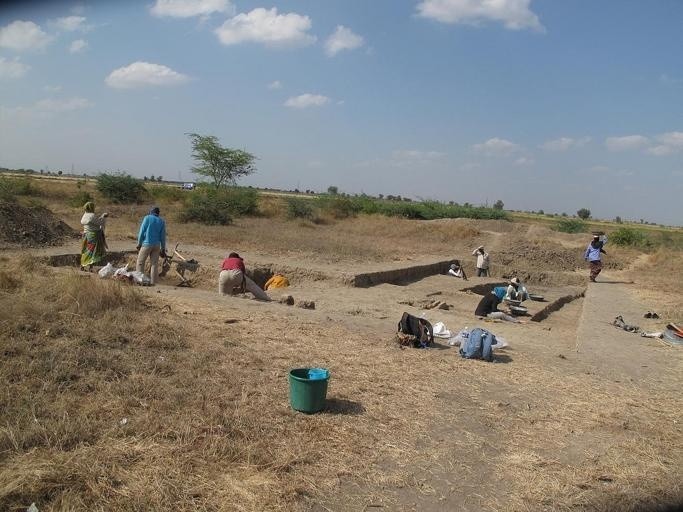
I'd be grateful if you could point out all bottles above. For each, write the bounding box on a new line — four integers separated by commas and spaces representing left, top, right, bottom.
461, 327, 468, 352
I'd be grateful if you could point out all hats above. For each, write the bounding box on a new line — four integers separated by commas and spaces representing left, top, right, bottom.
151, 208, 160, 213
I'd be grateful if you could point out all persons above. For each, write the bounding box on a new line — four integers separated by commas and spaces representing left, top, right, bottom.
80, 201, 109, 272
136, 206, 167, 285
446, 264, 462, 277
584, 234, 608, 282
474, 277, 530, 325
471, 245, 491, 277
218, 252, 272, 302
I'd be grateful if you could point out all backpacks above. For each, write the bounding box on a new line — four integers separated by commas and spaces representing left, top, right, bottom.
397, 311, 434, 348
459, 327, 497, 361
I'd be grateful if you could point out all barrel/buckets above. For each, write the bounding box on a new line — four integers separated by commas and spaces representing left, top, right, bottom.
494, 285, 509, 299
289, 368, 331, 412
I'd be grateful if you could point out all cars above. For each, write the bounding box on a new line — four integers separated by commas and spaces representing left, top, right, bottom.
180, 183, 196, 189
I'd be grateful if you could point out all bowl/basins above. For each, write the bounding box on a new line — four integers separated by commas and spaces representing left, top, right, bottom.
503, 298, 522, 307
511, 306, 529, 317
529, 293, 545, 302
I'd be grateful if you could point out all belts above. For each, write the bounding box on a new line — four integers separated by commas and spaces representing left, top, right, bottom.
222, 269, 233, 270
